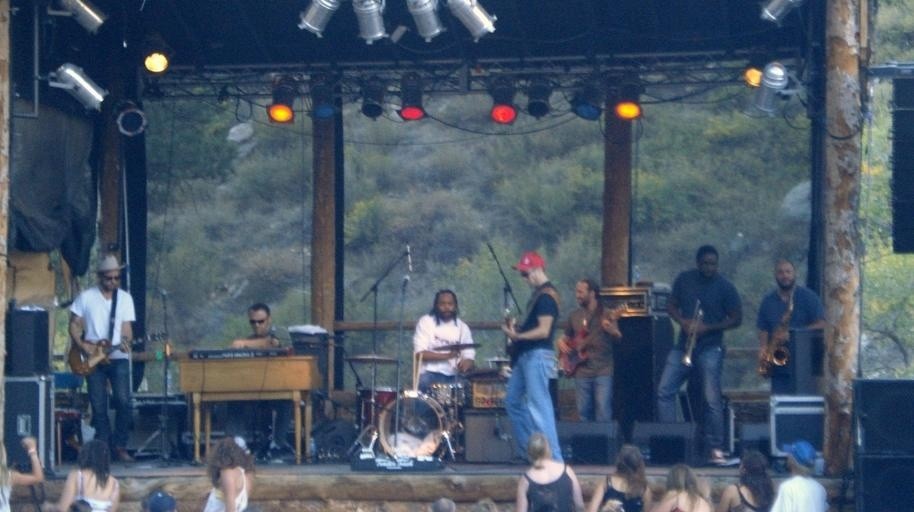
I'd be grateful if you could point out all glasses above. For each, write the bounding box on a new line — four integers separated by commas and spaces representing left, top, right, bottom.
249, 319, 264, 323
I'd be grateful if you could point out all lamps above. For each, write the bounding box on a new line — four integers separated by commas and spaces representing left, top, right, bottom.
743, 0, 810, 124
298, 1, 498, 51
9, 1, 109, 122
135, 39, 813, 139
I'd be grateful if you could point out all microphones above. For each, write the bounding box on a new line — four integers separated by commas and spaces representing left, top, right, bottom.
401, 275, 410, 289
407, 246, 412, 272
494, 413, 502, 435
479, 225, 491, 244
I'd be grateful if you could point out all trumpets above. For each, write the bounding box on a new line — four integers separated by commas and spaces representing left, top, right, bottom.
681, 299, 703, 368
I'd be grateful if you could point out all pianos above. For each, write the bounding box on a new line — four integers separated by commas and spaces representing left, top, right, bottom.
189, 347, 293, 360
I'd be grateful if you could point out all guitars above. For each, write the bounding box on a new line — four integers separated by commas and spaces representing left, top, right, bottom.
69, 331, 171, 377
559, 304, 628, 378
505, 308, 520, 368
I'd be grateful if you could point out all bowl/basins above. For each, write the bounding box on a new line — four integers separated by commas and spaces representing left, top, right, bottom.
291, 333, 326, 356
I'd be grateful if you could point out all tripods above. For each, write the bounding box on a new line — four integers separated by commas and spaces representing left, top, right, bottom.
341, 287, 380, 464
438, 353, 465, 463
133, 311, 190, 465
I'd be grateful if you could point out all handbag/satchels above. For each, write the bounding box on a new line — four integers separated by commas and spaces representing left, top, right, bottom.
69, 499, 92, 512
601, 499, 625, 512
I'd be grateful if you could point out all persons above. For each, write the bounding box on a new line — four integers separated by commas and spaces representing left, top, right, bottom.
232, 302, 292, 447
500, 252, 562, 467
412, 290, 476, 451
69, 256, 138, 462
0, 426, 257, 511
560, 279, 622, 423
657, 246, 742, 464
756, 258, 826, 394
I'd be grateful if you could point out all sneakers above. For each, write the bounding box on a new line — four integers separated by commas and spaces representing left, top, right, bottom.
113, 447, 133, 462
708, 449, 726, 464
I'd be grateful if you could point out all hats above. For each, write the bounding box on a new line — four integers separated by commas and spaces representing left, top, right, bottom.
784, 440, 816, 467
96, 255, 126, 272
511, 252, 544, 272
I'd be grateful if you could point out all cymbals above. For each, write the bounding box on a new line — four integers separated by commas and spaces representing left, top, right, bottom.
343, 353, 403, 364
433, 344, 482, 351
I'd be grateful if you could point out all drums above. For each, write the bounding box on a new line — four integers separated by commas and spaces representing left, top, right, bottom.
427, 383, 465, 407
379, 394, 448, 461
357, 386, 403, 422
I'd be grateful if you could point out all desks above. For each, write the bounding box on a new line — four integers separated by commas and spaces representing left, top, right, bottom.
178, 356, 321, 464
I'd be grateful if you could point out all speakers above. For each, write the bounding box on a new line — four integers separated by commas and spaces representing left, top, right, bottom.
892, 77, 914, 254
632, 420, 708, 465
854, 378, 914, 454
856, 454, 914, 512
556, 420, 624, 464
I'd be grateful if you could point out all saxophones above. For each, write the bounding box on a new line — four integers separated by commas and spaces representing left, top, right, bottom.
759, 286, 797, 374
